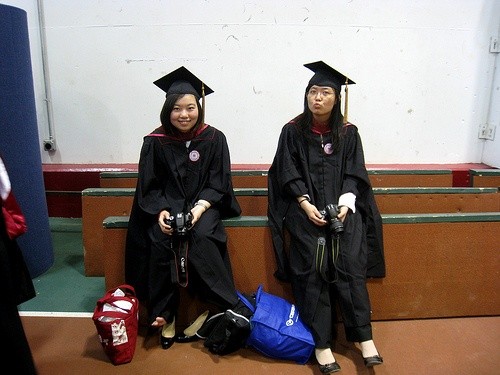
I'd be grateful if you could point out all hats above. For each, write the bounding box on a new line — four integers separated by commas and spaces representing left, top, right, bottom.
304, 61, 357, 124
154, 66, 215, 127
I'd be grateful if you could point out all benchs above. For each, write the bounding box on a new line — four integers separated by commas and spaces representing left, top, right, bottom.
100, 211, 500, 322
98, 170, 453, 189
468, 169, 500, 193
80, 188, 500, 278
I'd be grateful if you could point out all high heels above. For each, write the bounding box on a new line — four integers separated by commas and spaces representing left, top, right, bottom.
176, 311, 210, 343
361, 350, 383, 366
315, 347, 341, 374
161, 317, 177, 349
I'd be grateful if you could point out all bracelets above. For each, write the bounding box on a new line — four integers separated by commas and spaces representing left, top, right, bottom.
298, 199, 307, 207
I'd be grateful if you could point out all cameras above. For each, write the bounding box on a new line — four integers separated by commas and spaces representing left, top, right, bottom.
320, 203, 346, 237
165, 211, 193, 239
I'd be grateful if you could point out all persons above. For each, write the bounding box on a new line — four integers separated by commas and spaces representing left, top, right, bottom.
267, 61, 386, 375
124, 66, 243, 326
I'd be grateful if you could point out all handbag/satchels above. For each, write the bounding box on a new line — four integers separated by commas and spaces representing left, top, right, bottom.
237, 285, 315, 365
92, 285, 139, 365
203, 295, 256, 356
